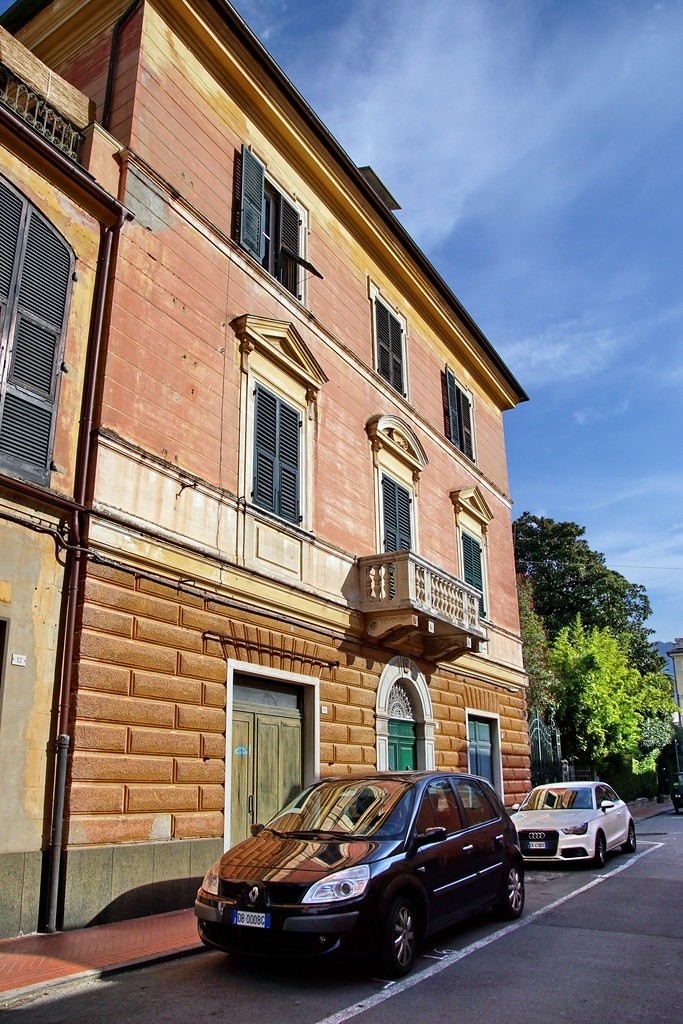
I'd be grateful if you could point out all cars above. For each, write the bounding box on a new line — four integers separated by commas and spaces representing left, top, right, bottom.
674, 739, 681, 773
671, 771, 683, 815
193, 769, 525, 979
511, 782, 638, 870
